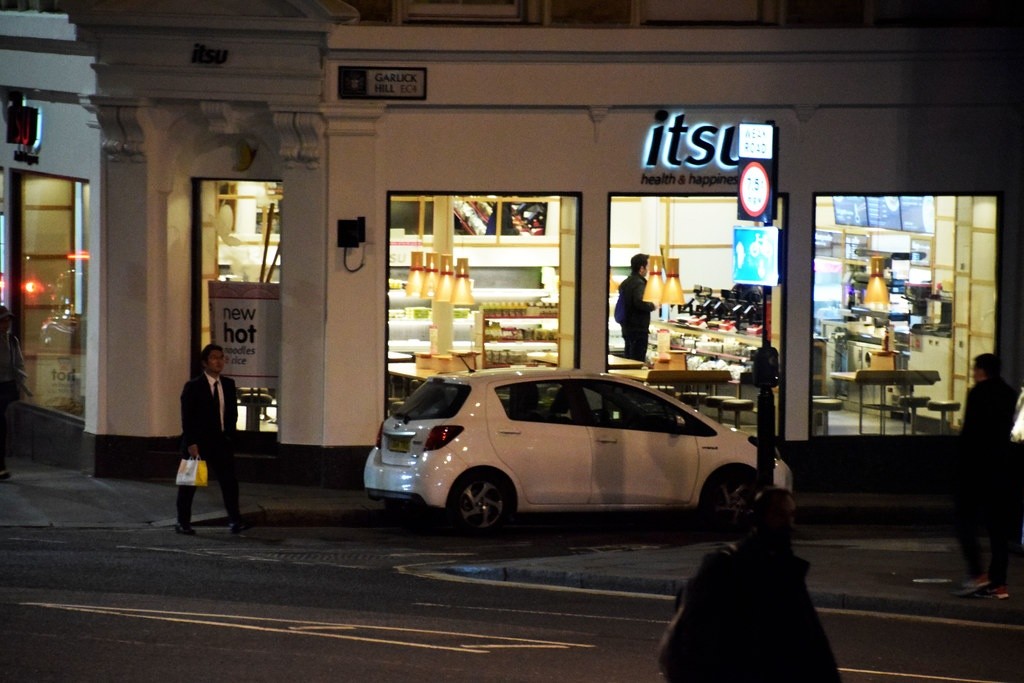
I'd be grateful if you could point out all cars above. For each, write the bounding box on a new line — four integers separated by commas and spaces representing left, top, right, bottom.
363, 367, 794, 537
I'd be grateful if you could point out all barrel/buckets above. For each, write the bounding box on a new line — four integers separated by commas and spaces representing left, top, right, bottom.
656, 329, 671, 353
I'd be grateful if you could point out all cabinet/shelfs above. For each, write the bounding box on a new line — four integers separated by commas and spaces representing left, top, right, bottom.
482, 308, 558, 368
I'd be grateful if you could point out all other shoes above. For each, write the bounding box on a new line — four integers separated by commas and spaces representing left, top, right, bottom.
987, 585, 1009, 599
231, 530, 247, 539
949, 574, 990, 596
0, 470, 10, 481
175, 525, 195, 536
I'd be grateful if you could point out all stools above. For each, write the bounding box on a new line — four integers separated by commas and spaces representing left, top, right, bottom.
928, 400, 961, 435
811, 396, 843, 437
650, 385, 754, 430
898, 396, 932, 435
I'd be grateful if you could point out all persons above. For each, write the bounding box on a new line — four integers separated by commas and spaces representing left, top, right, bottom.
174, 344, 253, 534
508, 382, 570, 423
485, 200, 520, 235
618, 254, 655, 361
947, 354, 1024, 599
651, 488, 847, 683
0, 305, 33, 479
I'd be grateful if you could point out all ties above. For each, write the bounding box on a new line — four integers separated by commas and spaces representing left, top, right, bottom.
214, 381, 222, 432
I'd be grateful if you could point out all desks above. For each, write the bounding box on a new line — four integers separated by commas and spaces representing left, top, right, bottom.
830, 370, 942, 435
527, 351, 644, 370
608, 369, 733, 414
388, 362, 438, 398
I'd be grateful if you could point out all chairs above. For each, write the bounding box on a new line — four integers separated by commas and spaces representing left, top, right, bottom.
546, 384, 597, 427
509, 382, 546, 421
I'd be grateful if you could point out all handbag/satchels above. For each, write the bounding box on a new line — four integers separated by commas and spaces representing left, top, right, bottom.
177, 456, 207, 486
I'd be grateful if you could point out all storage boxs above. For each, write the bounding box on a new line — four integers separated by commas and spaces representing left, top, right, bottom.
413, 351, 474, 372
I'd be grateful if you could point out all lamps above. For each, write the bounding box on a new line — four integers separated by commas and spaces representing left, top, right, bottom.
642, 198, 686, 305
406, 196, 475, 306
863, 197, 890, 305
335, 216, 365, 273
